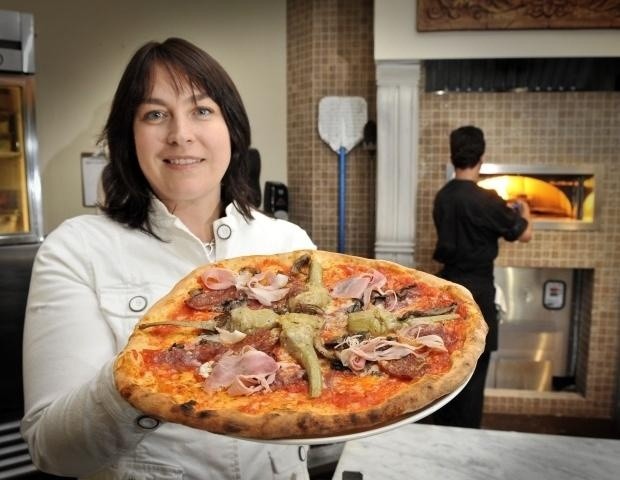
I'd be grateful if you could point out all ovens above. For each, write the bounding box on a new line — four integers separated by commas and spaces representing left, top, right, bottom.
454, 168, 590, 391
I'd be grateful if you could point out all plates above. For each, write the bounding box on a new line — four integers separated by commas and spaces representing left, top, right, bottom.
217, 370, 474, 445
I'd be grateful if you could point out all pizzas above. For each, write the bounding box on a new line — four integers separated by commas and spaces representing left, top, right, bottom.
113, 249, 489, 440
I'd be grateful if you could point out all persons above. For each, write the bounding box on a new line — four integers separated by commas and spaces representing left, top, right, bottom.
19, 35, 321, 480
427, 125, 533, 427
